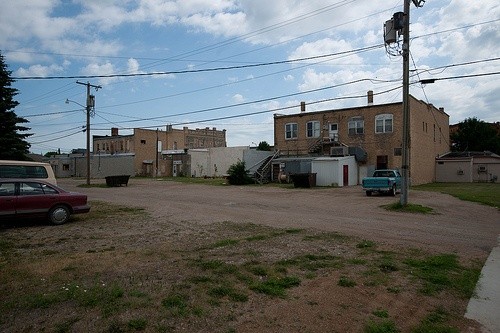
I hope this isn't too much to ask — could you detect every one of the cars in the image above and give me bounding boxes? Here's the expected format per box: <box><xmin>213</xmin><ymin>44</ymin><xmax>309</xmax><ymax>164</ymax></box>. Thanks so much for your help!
<box><xmin>0</xmin><ymin>180</ymin><xmax>92</xmax><ymax>225</ymax></box>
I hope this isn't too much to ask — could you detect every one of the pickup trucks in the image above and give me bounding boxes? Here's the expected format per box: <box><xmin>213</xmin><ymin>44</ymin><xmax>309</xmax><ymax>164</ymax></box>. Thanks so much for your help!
<box><xmin>361</xmin><ymin>170</ymin><xmax>403</xmax><ymax>196</ymax></box>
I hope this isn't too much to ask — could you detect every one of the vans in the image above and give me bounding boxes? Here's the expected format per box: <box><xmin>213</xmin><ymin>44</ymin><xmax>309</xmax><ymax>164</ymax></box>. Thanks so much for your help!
<box><xmin>0</xmin><ymin>161</ymin><xmax>58</xmax><ymax>187</ymax></box>
